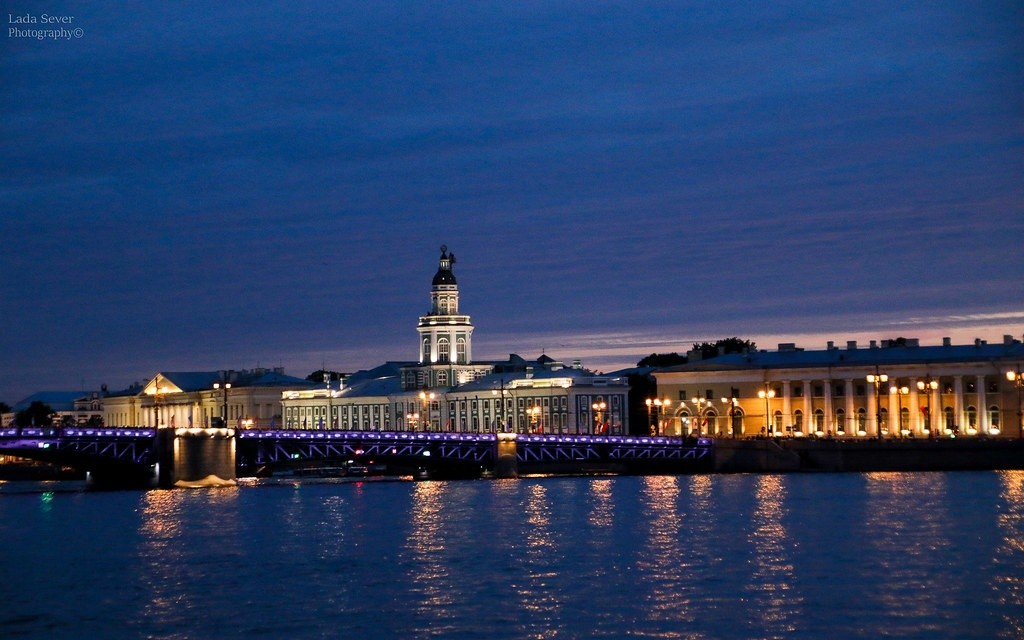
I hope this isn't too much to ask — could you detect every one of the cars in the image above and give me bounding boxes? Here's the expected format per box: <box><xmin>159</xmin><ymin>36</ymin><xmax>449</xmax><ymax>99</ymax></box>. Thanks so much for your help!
<box><xmin>338</xmin><ymin>454</ymin><xmax>376</xmax><ymax>478</ymax></box>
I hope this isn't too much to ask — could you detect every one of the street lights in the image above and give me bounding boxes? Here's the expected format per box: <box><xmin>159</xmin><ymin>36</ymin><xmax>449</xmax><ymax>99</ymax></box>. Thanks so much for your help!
<box><xmin>407</xmin><ymin>411</ymin><xmax>419</xmax><ymax>433</ymax></box>
<box><xmin>758</xmin><ymin>384</ymin><xmax>775</xmax><ymax>438</ymax></box>
<box><xmin>420</xmin><ymin>389</ymin><xmax>435</xmax><ymax>430</ymax></box>
<box><xmin>526</xmin><ymin>406</ymin><xmax>540</xmax><ymax>432</ymax></box>
<box><xmin>659</xmin><ymin>399</ymin><xmax>670</xmax><ymax>437</ymax></box>
<box><xmin>867</xmin><ymin>362</ymin><xmax>888</xmax><ymax>439</ymax></box>
<box><xmin>645</xmin><ymin>399</ymin><xmax>660</xmax><ymax>435</ymax></box>
<box><xmin>592</xmin><ymin>403</ymin><xmax>606</xmax><ymax>422</ymax></box>
<box><xmin>691</xmin><ymin>390</ymin><xmax>705</xmax><ymax>437</ymax></box>
<box><xmin>1006</xmin><ymin>362</ymin><xmax>1024</xmax><ymax>438</ymax></box>
<box><xmin>492</xmin><ymin>378</ymin><xmax>508</xmax><ymax>432</ymax></box>
<box><xmin>916</xmin><ymin>373</ymin><xmax>939</xmax><ymax>436</ymax></box>
<box><xmin>721</xmin><ymin>386</ymin><xmax>737</xmax><ymax>439</ymax></box>
<box><xmin>890</xmin><ymin>379</ymin><xmax>909</xmax><ymax>439</ymax></box>
<box><xmin>212</xmin><ymin>378</ymin><xmax>231</xmax><ymax>427</ymax></box>
